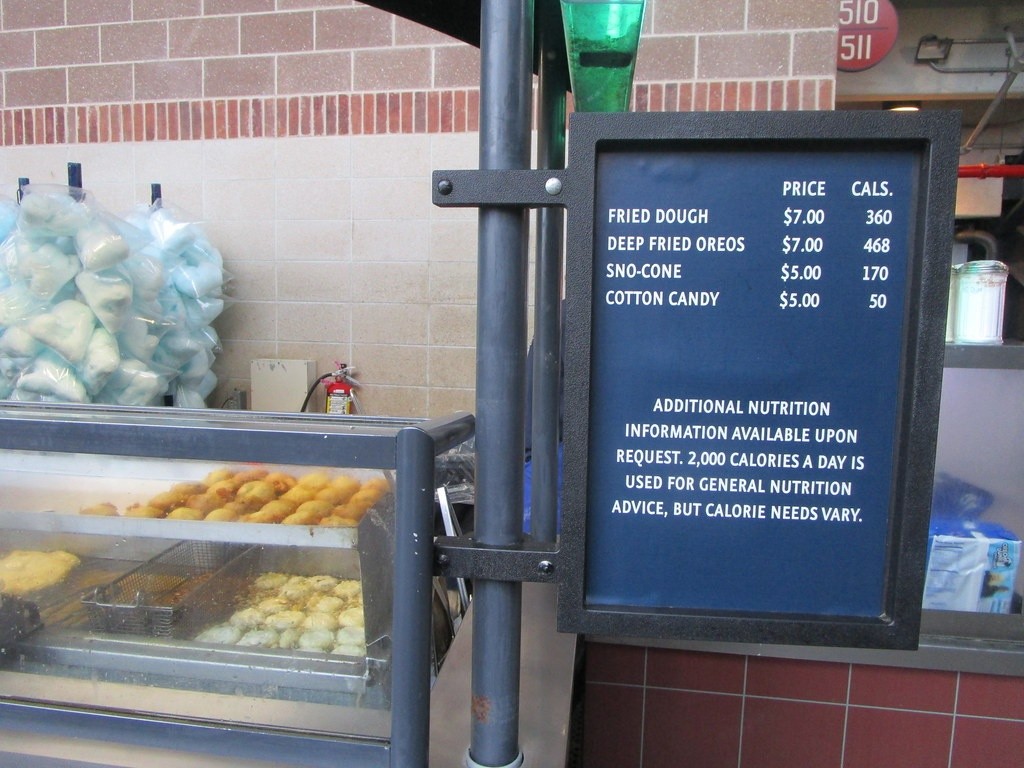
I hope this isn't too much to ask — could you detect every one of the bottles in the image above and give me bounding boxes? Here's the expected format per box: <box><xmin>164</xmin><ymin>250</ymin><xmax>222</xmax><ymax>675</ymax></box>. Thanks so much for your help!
<box><xmin>956</xmin><ymin>257</ymin><xmax>1009</xmax><ymax>344</ymax></box>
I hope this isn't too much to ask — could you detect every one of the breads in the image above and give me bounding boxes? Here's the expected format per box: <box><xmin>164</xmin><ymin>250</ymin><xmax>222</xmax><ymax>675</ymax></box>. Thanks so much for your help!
<box><xmin>0</xmin><ymin>196</ymin><xmax>225</xmax><ymax>403</ymax></box>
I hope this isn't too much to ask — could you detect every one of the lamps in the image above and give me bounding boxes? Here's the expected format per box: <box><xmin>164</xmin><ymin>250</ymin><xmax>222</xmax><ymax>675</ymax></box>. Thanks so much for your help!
<box><xmin>914</xmin><ymin>34</ymin><xmax>954</xmax><ymax>62</ymax></box>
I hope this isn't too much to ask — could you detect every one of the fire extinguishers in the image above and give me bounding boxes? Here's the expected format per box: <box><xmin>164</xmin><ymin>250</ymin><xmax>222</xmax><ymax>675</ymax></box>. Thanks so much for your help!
<box><xmin>301</xmin><ymin>360</ymin><xmax>359</xmax><ymax>414</ymax></box>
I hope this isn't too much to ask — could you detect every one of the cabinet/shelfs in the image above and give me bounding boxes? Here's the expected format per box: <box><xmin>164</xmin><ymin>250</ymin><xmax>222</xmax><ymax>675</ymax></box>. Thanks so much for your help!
<box><xmin>0</xmin><ymin>467</ymin><xmax>389</xmax><ymax>692</ymax></box>
